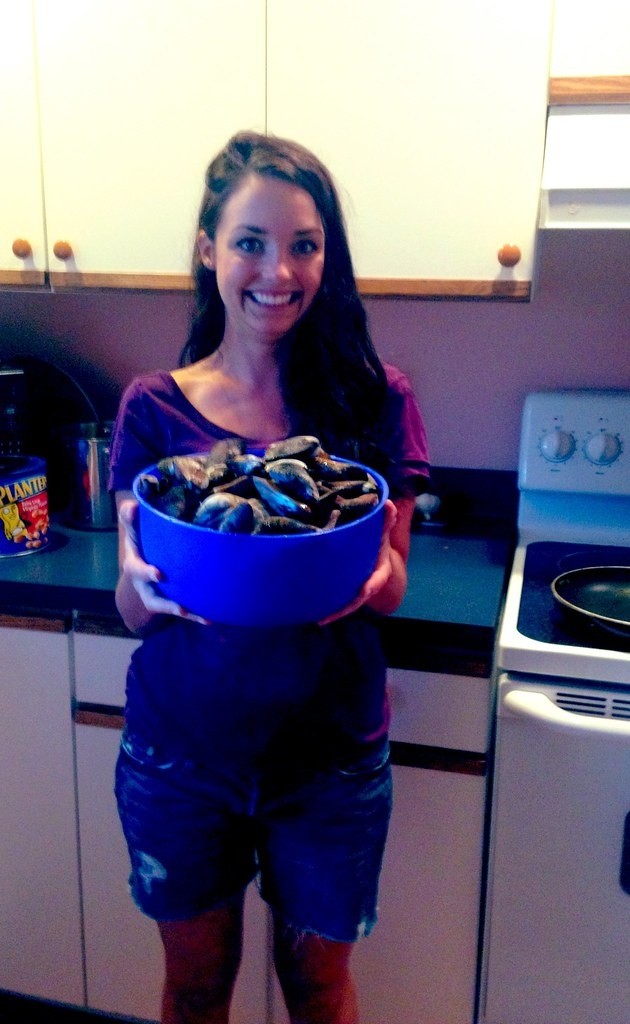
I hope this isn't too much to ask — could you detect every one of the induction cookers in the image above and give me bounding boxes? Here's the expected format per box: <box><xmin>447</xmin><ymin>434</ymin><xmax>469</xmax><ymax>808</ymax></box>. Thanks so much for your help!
<box><xmin>491</xmin><ymin>389</ymin><xmax>630</xmax><ymax>684</ymax></box>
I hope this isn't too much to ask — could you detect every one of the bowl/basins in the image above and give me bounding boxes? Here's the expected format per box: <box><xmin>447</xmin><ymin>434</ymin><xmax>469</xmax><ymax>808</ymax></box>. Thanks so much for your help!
<box><xmin>132</xmin><ymin>453</ymin><xmax>390</xmax><ymax>625</ymax></box>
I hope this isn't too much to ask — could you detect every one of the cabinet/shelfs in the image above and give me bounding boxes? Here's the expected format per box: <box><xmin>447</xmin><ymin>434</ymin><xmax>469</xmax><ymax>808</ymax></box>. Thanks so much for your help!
<box><xmin>0</xmin><ymin>0</ymin><xmax>556</xmax><ymax>298</ymax></box>
<box><xmin>0</xmin><ymin>608</ymin><xmax>492</xmax><ymax>1024</ymax></box>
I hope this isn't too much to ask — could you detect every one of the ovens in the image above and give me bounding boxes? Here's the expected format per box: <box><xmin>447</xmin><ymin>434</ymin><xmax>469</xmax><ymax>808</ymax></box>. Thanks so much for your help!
<box><xmin>478</xmin><ymin>672</ymin><xmax>630</xmax><ymax>1024</ymax></box>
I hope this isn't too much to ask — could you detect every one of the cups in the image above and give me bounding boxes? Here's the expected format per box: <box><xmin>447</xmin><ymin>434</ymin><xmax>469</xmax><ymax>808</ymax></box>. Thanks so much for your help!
<box><xmin>63</xmin><ymin>422</ymin><xmax>120</xmax><ymax>526</ymax></box>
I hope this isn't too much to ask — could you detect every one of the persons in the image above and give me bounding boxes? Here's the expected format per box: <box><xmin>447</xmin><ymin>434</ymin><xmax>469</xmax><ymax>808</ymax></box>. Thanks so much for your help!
<box><xmin>107</xmin><ymin>129</ymin><xmax>432</xmax><ymax>1024</ymax></box>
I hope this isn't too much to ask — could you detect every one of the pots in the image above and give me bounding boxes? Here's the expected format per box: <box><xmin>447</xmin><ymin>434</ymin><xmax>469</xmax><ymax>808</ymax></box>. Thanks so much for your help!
<box><xmin>549</xmin><ymin>552</ymin><xmax>630</xmax><ymax>634</ymax></box>
